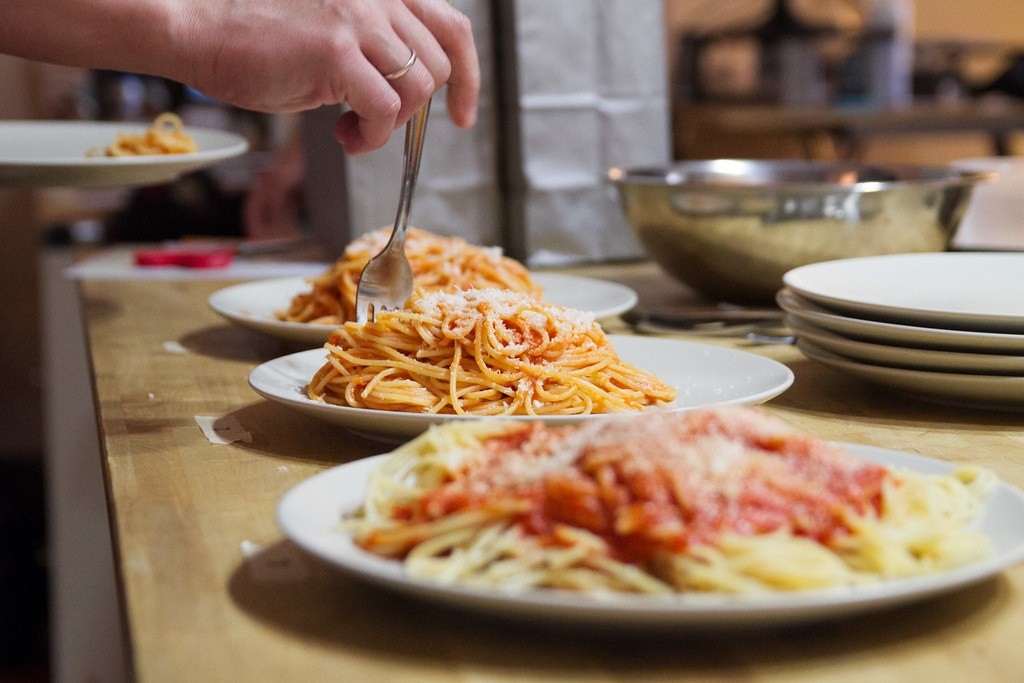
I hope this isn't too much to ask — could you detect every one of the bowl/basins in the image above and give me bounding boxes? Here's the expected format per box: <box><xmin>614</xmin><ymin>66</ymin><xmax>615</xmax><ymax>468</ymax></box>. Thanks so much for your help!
<box><xmin>598</xmin><ymin>162</ymin><xmax>998</xmax><ymax>298</ymax></box>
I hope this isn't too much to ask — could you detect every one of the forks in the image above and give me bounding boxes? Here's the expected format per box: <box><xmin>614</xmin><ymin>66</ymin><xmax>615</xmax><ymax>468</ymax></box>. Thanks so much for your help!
<box><xmin>357</xmin><ymin>97</ymin><xmax>429</xmax><ymax>322</ymax></box>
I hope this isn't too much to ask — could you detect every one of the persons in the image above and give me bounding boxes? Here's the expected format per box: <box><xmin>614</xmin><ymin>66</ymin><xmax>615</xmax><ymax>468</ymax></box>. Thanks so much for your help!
<box><xmin>2</xmin><ymin>0</ymin><xmax>480</xmax><ymax>158</ymax></box>
<box><xmin>134</xmin><ymin>75</ymin><xmax>247</xmax><ymax>240</ymax></box>
<box><xmin>247</xmin><ymin>1</ymin><xmax>674</xmax><ymax>265</ymax></box>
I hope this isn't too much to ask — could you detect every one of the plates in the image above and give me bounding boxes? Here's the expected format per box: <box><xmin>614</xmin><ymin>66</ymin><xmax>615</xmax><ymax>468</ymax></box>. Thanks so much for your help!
<box><xmin>275</xmin><ymin>439</ymin><xmax>1023</xmax><ymax>630</ymax></box>
<box><xmin>206</xmin><ymin>269</ymin><xmax>640</xmax><ymax>349</ymax></box>
<box><xmin>249</xmin><ymin>336</ymin><xmax>796</xmax><ymax>439</ymax></box>
<box><xmin>777</xmin><ymin>254</ymin><xmax>1023</xmax><ymax>401</ymax></box>
<box><xmin>1</xmin><ymin>120</ymin><xmax>248</xmax><ymax>188</ymax></box>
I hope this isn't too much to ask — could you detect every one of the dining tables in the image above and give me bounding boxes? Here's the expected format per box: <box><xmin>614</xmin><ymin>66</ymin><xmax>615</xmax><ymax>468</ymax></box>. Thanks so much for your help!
<box><xmin>36</xmin><ymin>236</ymin><xmax>1023</xmax><ymax>683</ymax></box>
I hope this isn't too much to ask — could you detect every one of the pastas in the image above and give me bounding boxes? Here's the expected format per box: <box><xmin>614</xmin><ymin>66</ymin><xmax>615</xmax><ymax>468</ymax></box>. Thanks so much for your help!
<box><xmin>307</xmin><ymin>282</ymin><xmax>673</xmax><ymax>419</ymax></box>
<box><xmin>102</xmin><ymin>112</ymin><xmax>197</xmax><ymax>159</ymax></box>
<box><xmin>277</xmin><ymin>222</ymin><xmax>539</xmax><ymax>325</ymax></box>
<box><xmin>344</xmin><ymin>413</ymin><xmax>991</xmax><ymax>600</ymax></box>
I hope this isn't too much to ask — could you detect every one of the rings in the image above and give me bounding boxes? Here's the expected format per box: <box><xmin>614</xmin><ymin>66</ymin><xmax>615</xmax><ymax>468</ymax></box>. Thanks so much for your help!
<box><xmin>382</xmin><ymin>46</ymin><xmax>417</xmax><ymax>80</ymax></box>
